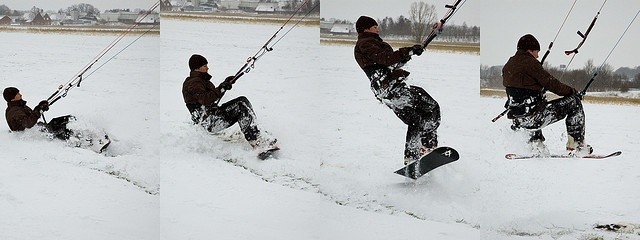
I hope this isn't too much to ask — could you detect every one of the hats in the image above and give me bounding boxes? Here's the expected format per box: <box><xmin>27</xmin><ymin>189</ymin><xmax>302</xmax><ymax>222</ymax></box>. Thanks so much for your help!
<box><xmin>3</xmin><ymin>87</ymin><xmax>19</xmax><ymax>102</ymax></box>
<box><xmin>356</xmin><ymin>16</ymin><xmax>378</xmax><ymax>34</ymax></box>
<box><xmin>189</xmin><ymin>55</ymin><xmax>208</xmax><ymax>72</ymax></box>
<box><xmin>517</xmin><ymin>34</ymin><xmax>540</xmax><ymax>50</ymax></box>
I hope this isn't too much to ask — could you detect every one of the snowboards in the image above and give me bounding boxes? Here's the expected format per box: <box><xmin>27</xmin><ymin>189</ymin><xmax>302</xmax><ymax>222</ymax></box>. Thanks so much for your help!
<box><xmin>86</xmin><ymin>134</ymin><xmax>112</xmax><ymax>152</ymax></box>
<box><xmin>258</xmin><ymin>139</ymin><xmax>280</xmax><ymax>160</ymax></box>
<box><xmin>394</xmin><ymin>146</ymin><xmax>460</xmax><ymax>179</ymax></box>
<box><xmin>505</xmin><ymin>151</ymin><xmax>621</xmax><ymax>159</ymax></box>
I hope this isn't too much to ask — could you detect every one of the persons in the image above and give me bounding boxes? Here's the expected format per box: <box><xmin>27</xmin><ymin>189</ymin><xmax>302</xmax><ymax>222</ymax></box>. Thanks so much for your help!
<box><xmin>354</xmin><ymin>16</ymin><xmax>441</xmax><ymax>165</ymax></box>
<box><xmin>3</xmin><ymin>87</ymin><xmax>92</xmax><ymax>148</ymax></box>
<box><xmin>182</xmin><ymin>54</ymin><xmax>280</xmax><ymax>160</ymax></box>
<box><xmin>502</xmin><ymin>34</ymin><xmax>593</xmax><ymax>157</ymax></box>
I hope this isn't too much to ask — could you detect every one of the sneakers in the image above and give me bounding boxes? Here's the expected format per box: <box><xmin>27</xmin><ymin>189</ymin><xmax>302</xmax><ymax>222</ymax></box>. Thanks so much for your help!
<box><xmin>568</xmin><ymin>142</ymin><xmax>593</xmax><ymax>155</ymax></box>
<box><xmin>532</xmin><ymin>140</ymin><xmax>550</xmax><ymax>155</ymax></box>
<box><xmin>404</xmin><ymin>158</ymin><xmax>414</xmax><ymax>165</ymax></box>
<box><xmin>419</xmin><ymin>146</ymin><xmax>433</xmax><ymax>156</ymax></box>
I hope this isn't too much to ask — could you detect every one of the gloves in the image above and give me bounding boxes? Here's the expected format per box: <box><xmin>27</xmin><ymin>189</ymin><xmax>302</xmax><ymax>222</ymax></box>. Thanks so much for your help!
<box><xmin>219</xmin><ymin>83</ymin><xmax>232</xmax><ymax>90</ymax></box>
<box><xmin>225</xmin><ymin>76</ymin><xmax>235</xmax><ymax>84</ymax></box>
<box><xmin>35</xmin><ymin>101</ymin><xmax>48</xmax><ymax>112</ymax></box>
<box><xmin>399</xmin><ymin>45</ymin><xmax>423</xmax><ymax>59</ymax></box>
